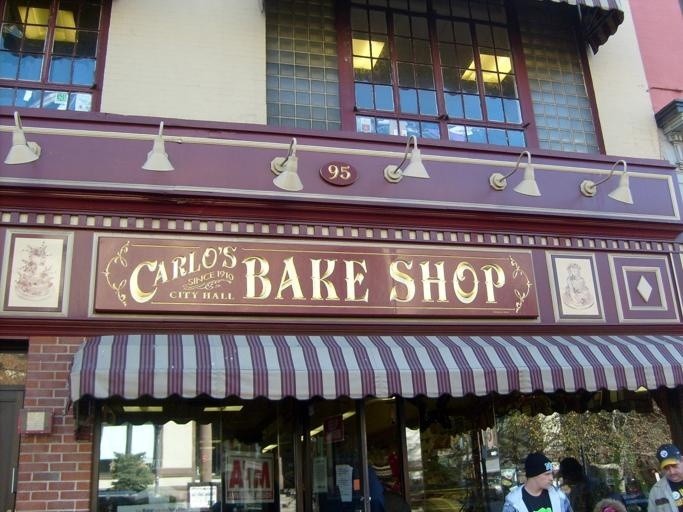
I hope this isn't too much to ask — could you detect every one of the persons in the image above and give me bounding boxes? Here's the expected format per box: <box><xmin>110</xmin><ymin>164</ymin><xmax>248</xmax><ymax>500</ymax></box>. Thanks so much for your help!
<box><xmin>557</xmin><ymin>456</ymin><xmax>608</xmax><ymax>511</ymax></box>
<box><xmin>502</xmin><ymin>449</ymin><xmax>573</xmax><ymax>511</ymax></box>
<box><xmin>335</xmin><ymin>434</ymin><xmax>383</xmax><ymax>512</ymax></box>
<box><xmin>199</xmin><ymin>470</ymin><xmax>243</xmax><ymax>511</ymax></box>
<box><xmin>648</xmin><ymin>443</ymin><xmax>683</xmax><ymax>510</ymax></box>
<box><xmin>129</xmin><ymin>468</ymin><xmax>186</xmax><ymax>511</ymax></box>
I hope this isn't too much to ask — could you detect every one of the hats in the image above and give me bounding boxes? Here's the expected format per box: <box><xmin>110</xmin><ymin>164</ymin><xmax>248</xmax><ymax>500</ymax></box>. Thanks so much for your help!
<box><xmin>525</xmin><ymin>453</ymin><xmax>553</xmax><ymax>478</ymax></box>
<box><xmin>657</xmin><ymin>444</ymin><xmax>681</xmax><ymax>469</ymax></box>
<box><xmin>555</xmin><ymin>457</ymin><xmax>582</xmax><ymax>478</ymax></box>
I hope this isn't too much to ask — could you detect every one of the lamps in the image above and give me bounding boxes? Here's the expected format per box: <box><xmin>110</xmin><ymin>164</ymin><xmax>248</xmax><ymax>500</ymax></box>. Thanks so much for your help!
<box><xmin>581</xmin><ymin>159</ymin><xmax>634</xmax><ymax>206</ymax></box>
<box><xmin>383</xmin><ymin>135</ymin><xmax>431</xmax><ymax>183</ymax></box>
<box><xmin>270</xmin><ymin>138</ymin><xmax>303</xmax><ymax>192</ymax></box>
<box><xmin>142</xmin><ymin>120</ymin><xmax>174</xmax><ymax>172</ymax></box>
<box><xmin>489</xmin><ymin>151</ymin><xmax>542</xmax><ymax>197</ymax></box>
<box><xmin>4</xmin><ymin>110</ymin><xmax>41</xmax><ymax>165</ymax></box>
